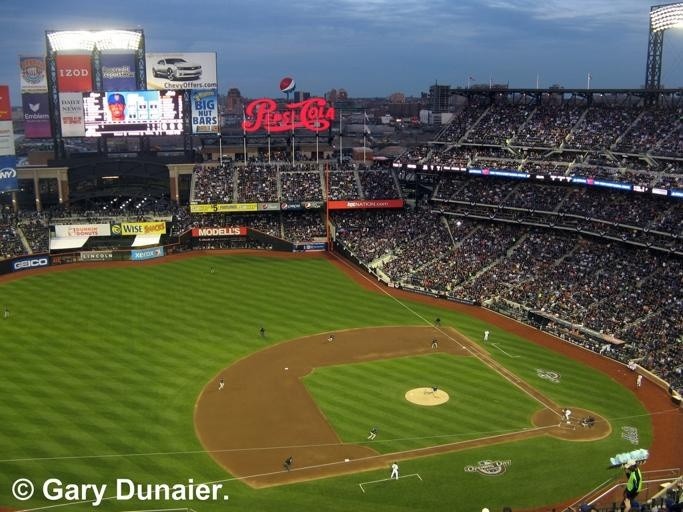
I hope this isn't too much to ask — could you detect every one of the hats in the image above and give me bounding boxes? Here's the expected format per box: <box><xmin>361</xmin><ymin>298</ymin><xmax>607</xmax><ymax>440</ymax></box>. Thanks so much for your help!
<box><xmin>581</xmin><ymin>503</ymin><xmax>591</xmax><ymax>512</ymax></box>
<box><xmin>625</xmin><ymin>460</ymin><xmax>635</xmax><ymax>468</ymax></box>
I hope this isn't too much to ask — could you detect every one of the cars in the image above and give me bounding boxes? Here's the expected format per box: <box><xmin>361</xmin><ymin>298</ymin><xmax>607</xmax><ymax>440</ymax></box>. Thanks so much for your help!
<box><xmin>152</xmin><ymin>55</ymin><xmax>203</xmax><ymax>82</ymax></box>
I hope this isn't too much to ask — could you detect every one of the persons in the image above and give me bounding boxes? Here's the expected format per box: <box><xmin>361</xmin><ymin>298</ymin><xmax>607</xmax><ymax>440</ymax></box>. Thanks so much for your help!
<box><xmin>107</xmin><ymin>94</ymin><xmax>125</xmax><ymax>120</ymax></box>
<box><xmin>284</xmin><ymin>455</ymin><xmax>295</xmax><ymax>471</ymax></box>
<box><xmin>558</xmin><ymin>408</ymin><xmax>576</xmax><ymax>431</ymax></box>
<box><xmin>587</xmin><ymin>508</ymin><xmax>599</xmax><ymax>512</ymax></box>
<box><xmin>368</xmin><ymin>428</ymin><xmax>377</xmax><ymax>440</ymax></box>
<box><xmin>665</xmin><ymin>479</ymin><xmax>683</xmax><ymax>512</ymax></box>
<box><xmin>503</xmin><ymin>506</ymin><xmax>512</xmax><ymax>512</ymax></box>
<box><xmin>621</xmin><ymin>459</ymin><xmax>643</xmax><ymax>505</ymax></box>
<box><xmin>390</xmin><ymin>461</ymin><xmax>400</xmax><ymax>480</ymax></box>
<box><xmin>623</xmin><ymin>498</ymin><xmax>632</xmax><ymax>512</ymax></box>
<box><xmin>481</xmin><ymin>507</ymin><xmax>490</xmax><ymax>512</ymax></box>
